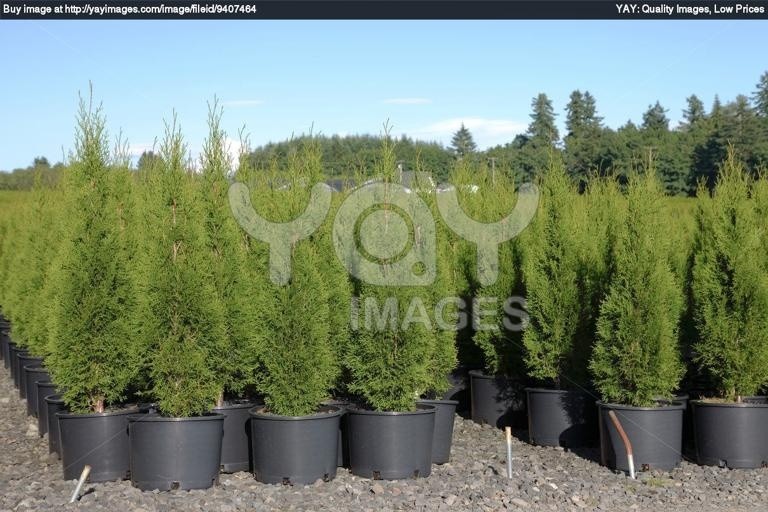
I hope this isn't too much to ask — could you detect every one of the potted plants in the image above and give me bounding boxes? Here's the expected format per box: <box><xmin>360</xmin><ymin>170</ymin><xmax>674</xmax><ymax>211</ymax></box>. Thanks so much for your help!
<box><xmin>0</xmin><ymin>76</ymin><xmax>768</xmax><ymax>494</ymax></box>
